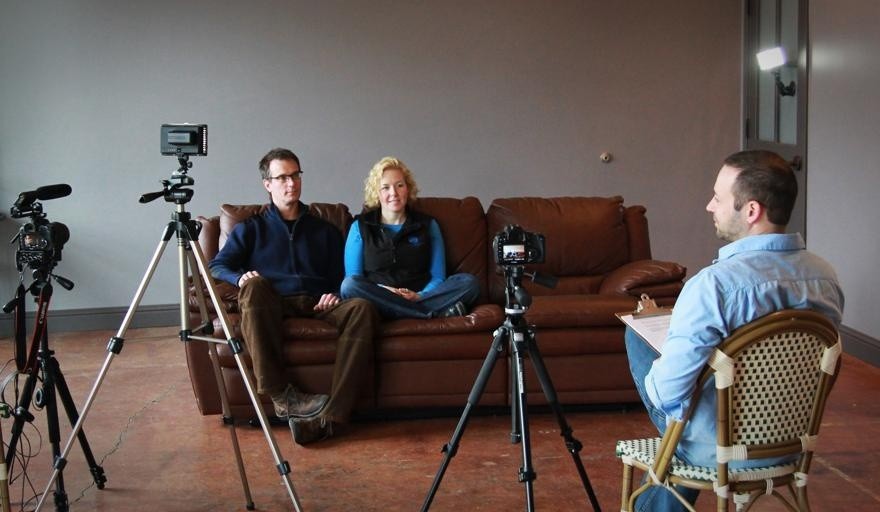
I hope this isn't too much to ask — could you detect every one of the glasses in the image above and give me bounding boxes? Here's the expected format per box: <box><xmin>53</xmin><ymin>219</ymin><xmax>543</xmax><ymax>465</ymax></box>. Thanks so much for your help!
<box><xmin>268</xmin><ymin>171</ymin><xmax>302</xmax><ymax>181</ymax></box>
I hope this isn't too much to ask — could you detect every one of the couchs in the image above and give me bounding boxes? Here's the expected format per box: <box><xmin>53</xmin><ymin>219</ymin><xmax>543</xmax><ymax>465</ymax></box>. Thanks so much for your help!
<box><xmin>174</xmin><ymin>190</ymin><xmax>689</xmax><ymax>432</ymax></box>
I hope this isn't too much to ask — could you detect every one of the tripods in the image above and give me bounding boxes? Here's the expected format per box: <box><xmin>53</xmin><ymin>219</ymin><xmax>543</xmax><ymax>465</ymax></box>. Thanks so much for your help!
<box><xmin>0</xmin><ymin>279</ymin><xmax>107</xmax><ymax>512</ymax></box>
<box><xmin>421</xmin><ymin>317</ymin><xmax>602</xmax><ymax>512</ymax></box>
<box><xmin>34</xmin><ymin>205</ymin><xmax>302</xmax><ymax>511</ymax></box>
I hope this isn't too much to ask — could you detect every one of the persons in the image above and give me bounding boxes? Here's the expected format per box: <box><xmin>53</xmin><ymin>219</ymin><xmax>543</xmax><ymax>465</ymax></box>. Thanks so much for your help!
<box><xmin>205</xmin><ymin>146</ymin><xmax>382</xmax><ymax>447</ymax></box>
<box><xmin>622</xmin><ymin>148</ymin><xmax>848</xmax><ymax>512</ymax></box>
<box><xmin>338</xmin><ymin>155</ymin><xmax>480</xmax><ymax>320</ymax></box>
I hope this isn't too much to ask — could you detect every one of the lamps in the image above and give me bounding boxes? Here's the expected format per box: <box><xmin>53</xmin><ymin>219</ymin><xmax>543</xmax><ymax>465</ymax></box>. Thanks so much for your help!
<box><xmin>754</xmin><ymin>41</ymin><xmax>798</xmax><ymax>102</ymax></box>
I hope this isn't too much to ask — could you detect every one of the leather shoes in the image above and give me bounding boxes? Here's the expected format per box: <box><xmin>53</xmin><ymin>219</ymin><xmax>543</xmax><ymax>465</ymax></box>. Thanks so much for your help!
<box><xmin>435</xmin><ymin>301</ymin><xmax>466</xmax><ymax>318</ymax></box>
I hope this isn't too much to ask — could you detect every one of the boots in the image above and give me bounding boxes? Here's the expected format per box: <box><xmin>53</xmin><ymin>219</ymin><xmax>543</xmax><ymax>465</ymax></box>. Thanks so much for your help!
<box><xmin>288</xmin><ymin>415</ymin><xmax>333</xmax><ymax>443</ymax></box>
<box><xmin>270</xmin><ymin>387</ymin><xmax>329</xmax><ymax>422</ymax></box>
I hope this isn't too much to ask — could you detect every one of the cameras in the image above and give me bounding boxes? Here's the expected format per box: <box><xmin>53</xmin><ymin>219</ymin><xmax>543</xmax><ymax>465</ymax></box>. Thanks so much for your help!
<box><xmin>492</xmin><ymin>223</ymin><xmax>546</xmax><ymax>267</ymax></box>
<box><xmin>16</xmin><ymin>223</ymin><xmax>70</xmax><ymax>268</ymax></box>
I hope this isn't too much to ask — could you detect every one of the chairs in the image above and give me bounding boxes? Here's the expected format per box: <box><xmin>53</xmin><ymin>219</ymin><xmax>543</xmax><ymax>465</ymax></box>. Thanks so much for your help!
<box><xmin>615</xmin><ymin>301</ymin><xmax>843</xmax><ymax>512</ymax></box>
